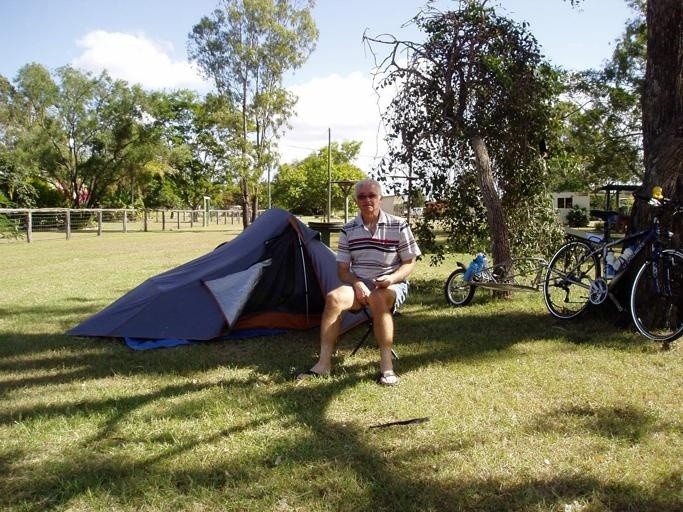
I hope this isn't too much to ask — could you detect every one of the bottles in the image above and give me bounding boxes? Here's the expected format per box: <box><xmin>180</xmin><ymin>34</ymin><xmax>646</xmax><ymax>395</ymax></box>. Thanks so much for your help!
<box><xmin>605</xmin><ymin>247</ymin><xmax>615</xmax><ymax>279</ymax></box>
<box><xmin>612</xmin><ymin>245</ymin><xmax>635</xmax><ymax>271</ymax></box>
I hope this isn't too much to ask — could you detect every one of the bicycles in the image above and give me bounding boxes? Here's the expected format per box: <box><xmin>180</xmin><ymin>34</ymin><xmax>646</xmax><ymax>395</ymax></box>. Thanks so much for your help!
<box><xmin>542</xmin><ymin>184</ymin><xmax>683</xmax><ymax>343</ymax></box>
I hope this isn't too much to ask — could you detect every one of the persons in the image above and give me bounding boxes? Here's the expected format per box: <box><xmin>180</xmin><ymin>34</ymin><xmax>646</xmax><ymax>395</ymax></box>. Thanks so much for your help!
<box><xmin>296</xmin><ymin>180</ymin><xmax>421</xmax><ymax>386</ymax></box>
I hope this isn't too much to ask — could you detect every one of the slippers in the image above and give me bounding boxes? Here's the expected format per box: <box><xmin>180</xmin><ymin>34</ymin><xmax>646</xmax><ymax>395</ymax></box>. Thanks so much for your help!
<box><xmin>295</xmin><ymin>370</ymin><xmax>322</xmax><ymax>381</ymax></box>
<box><xmin>380</xmin><ymin>372</ymin><xmax>399</xmax><ymax>387</ymax></box>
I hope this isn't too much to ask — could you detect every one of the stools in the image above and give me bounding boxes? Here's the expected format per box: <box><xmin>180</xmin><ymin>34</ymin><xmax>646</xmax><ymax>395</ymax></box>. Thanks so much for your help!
<box><xmin>348</xmin><ymin>303</ymin><xmax>399</xmax><ymax>362</ymax></box>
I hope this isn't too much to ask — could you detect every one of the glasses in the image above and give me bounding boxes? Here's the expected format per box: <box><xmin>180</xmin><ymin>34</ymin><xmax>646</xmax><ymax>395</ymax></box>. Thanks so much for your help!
<box><xmin>357</xmin><ymin>193</ymin><xmax>379</xmax><ymax>200</ymax></box>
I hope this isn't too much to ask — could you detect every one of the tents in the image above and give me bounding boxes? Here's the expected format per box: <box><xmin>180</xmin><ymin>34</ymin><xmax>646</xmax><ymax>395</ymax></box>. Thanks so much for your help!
<box><xmin>66</xmin><ymin>207</ymin><xmax>373</xmax><ymax>351</ymax></box>
